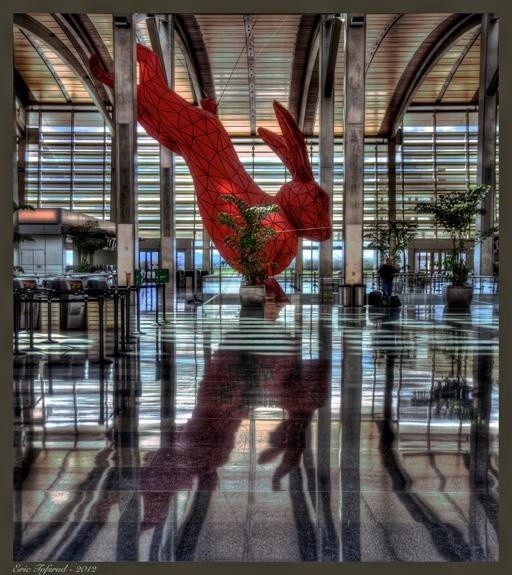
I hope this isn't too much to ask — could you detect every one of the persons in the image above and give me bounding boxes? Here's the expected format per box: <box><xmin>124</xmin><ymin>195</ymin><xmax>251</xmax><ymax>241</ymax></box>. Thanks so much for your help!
<box><xmin>376</xmin><ymin>258</ymin><xmax>398</xmax><ymax>308</ymax></box>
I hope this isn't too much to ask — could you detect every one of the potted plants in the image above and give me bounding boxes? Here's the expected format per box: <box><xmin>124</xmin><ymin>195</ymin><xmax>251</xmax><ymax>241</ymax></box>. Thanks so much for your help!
<box><xmin>212</xmin><ymin>193</ymin><xmax>281</xmax><ymax>308</ymax></box>
<box><xmin>412</xmin><ymin>183</ymin><xmax>499</xmax><ymax>312</ymax></box>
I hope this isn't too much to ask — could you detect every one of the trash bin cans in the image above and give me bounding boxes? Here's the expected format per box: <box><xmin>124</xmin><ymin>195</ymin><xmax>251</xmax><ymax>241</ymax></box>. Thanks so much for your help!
<box><xmin>338</xmin><ymin>284</ymin><xmax>366</xmax><ymax>307</ymax></box>
